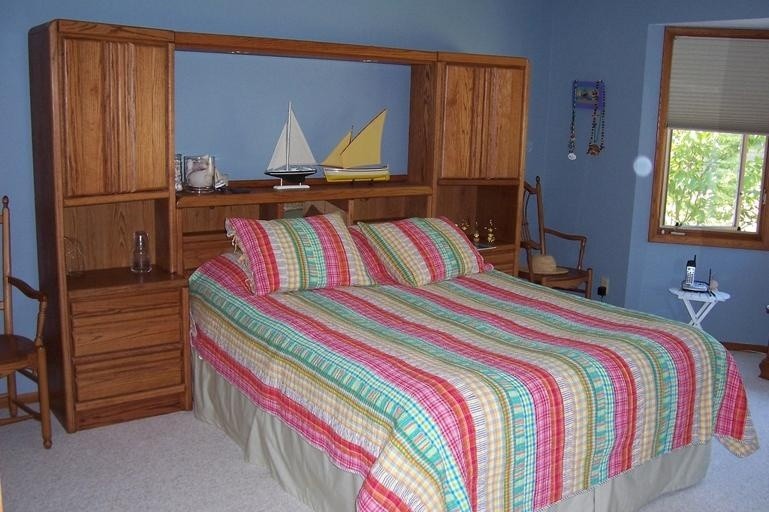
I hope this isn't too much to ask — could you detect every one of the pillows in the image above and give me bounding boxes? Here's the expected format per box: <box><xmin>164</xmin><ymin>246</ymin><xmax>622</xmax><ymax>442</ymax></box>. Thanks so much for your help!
<box><xmin>354</xmin><ymin>214</ymin><xmax>496</xmax><ymax>290</ymax></box>
<box><xmin>221</xmin><ymin>210</ymin><xmax>379</xmax><ymax>298</ymax></box>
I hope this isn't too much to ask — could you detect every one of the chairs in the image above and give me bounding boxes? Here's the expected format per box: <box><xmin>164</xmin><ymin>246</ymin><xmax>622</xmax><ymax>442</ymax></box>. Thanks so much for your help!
<box><xmin>518</xmin><ymin>175</ymin><xmax>593</xmax><ymax>300</ymax></box>
<box><xmin>0</xmin><ymin>193</ymin><xmax>53</xmax><ymax>449</ymax></box>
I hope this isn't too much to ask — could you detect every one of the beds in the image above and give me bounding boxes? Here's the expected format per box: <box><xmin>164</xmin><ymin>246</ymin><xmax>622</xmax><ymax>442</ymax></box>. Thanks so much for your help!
<box><xmin>188</xmin><ymin>248</ymin><xmax>722</xmax><ymax>510</ymax></box>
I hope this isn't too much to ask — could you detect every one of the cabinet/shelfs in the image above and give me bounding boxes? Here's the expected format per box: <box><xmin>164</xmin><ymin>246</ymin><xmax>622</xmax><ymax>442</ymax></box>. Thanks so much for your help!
<box><xmin>173</xmin><ymin>29</ymin><xmax>443</xmax><ymax>270</ymax></box>
<box><xmin>28</xmin><ymin>20</ymin><xmax>190</xmax><ymax>432</ymax></box>
<box><xmin>434</xmin><ymin>47</ymin><xmax>522</xmax><ymax>276</ymax></box>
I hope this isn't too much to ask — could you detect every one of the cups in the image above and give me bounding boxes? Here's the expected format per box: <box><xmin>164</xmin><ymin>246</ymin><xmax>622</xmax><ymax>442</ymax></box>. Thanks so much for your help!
<box><xmin>183</xmin><ymin>155</ymin><xmax>215</xmax><ymax>193</ymax></box>
<box><xmin>133</xmin><ymin>231</ymin><xmax>150</xmax><ymax>272</ymax></box>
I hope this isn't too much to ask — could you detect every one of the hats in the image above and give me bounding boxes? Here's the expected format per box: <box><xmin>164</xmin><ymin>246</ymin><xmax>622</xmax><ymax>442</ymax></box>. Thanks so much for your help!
<box><xmin>519</xmin><ymin>254</ymin><xmax>571</xmax><ymax>275</ymax></box>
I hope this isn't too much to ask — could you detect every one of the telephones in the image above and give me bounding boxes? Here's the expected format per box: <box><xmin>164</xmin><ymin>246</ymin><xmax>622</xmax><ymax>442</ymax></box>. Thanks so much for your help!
<box><xmin>682</xmin><ymin>255</ymin><xmax>709</xmax><ymax>293</ymax></box>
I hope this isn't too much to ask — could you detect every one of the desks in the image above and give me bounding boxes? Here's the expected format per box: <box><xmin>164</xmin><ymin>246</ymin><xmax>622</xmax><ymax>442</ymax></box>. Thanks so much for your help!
<box><xmin>669</xmin><ymin>284</ymin><xmax>730</xmax><ymax>333</ymax></box>
